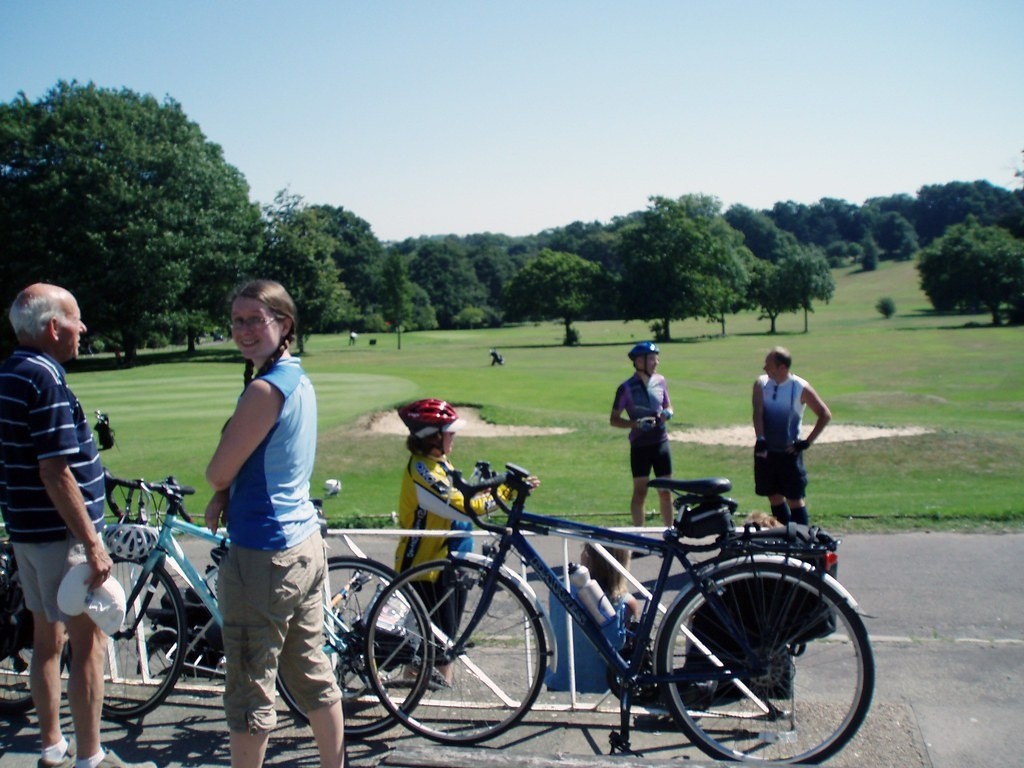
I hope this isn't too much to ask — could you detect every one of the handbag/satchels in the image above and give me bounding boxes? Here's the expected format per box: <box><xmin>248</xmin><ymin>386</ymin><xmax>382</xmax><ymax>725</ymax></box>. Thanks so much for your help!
<box><xmin>725</xmin><ymin>522</ymin><xmax>838</xmax><ymax>656</ymax></box>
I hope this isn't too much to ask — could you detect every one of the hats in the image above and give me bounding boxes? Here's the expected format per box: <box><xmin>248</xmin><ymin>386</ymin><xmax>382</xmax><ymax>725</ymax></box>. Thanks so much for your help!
<box><xmin>57</xmin><ymin>561</ymin><xmax>126</xmax><ymax>636</ymax></box>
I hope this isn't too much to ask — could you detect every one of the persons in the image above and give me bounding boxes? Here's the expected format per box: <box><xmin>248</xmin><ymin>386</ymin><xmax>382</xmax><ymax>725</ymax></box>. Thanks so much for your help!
<box><xmin>350</xmin><ymin>330</ymin><xmax>357</xmax><ymax>345</ymax></box>
<box><xmin>490</xmin><ymin>351</ymin><xmax>503</xmax><ymax>365</ymax></box>
<box><xmin>394</xmin><ymin>399</ymin><xmax>541</xmax><ymax>687</ymax></box>
<box><xmin>609</xmin><ymin>342</ymin><xmax>675</xmax><ymax>527</ymax></box>
<box><xmin>0</xmin><ymin>282</ymin><xmax>156</xmax><ymax>768</ymax></box>
<box><xmin>204</xmin><ymin>280</ymin><xmax>346</xmax><ymax>768</ymax></box>
<box><xmin>579</xmin><ymin>544</ymin><xmax>640</xmax><ymax>622</ymax></box>
<box><xmin>741</xmin><ymin>510</ymin><xmax>784</xmax><ymax>528</ymax></box>
<box><xmin>753</xmin><ymin>347</ymin><xmax>832</xmax><ymax>526</ymax></box>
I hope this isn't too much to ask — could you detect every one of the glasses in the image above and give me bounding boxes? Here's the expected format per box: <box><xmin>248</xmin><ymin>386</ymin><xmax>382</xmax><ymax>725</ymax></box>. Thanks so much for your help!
<box><xmin>232</xmin><ymin>316</ymin><xmax>277</xmax><ymax>328</ymax></box>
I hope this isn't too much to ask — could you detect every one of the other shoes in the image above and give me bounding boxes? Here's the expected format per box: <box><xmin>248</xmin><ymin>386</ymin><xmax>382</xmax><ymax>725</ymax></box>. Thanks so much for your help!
<box><xmin>403</xmin><ymin>666</ymin><xmax>418</xmax><ymax>679</ymax></box>
<box><xmin>72</xmin><ymin>749</ymin><xmax>157</xmax><ymax>768</ymax></box>
<box><xmin>37</xmin><ymin>735</ymin><xmax>76</xmax><ymax>768</ymax></box>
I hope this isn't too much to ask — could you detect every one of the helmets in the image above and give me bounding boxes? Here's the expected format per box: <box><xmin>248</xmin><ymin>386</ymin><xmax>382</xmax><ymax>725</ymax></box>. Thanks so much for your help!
<box><xmin>102</xmin><ymin>523</ymin><xmax>160</xmax><ymax>560</ymax></box>
<box><xmin>628</xmin><ymin>340</ymin><xmax>660</xmax><ymax>360</ymax></box>
<box><xmin>397</xmin><ymin>398</ymin><xmax>459</xmax><ymax>428</ymax></box>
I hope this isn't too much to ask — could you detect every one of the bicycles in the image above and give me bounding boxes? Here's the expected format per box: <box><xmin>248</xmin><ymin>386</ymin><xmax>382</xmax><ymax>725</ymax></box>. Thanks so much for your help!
<box><xmin>362</xmin><ymin>459</ymin><xmax>874</xmax><ymax>765</ymax></box>
<box><xmin>68</xmin><ymin>466</ymin><xmax>434</xmax><ymax>737</ymax></box>
<box><xmin>0</xmin><ymin>542</ymin><xmax>64</xmax><ymax>714</ymax></box>
